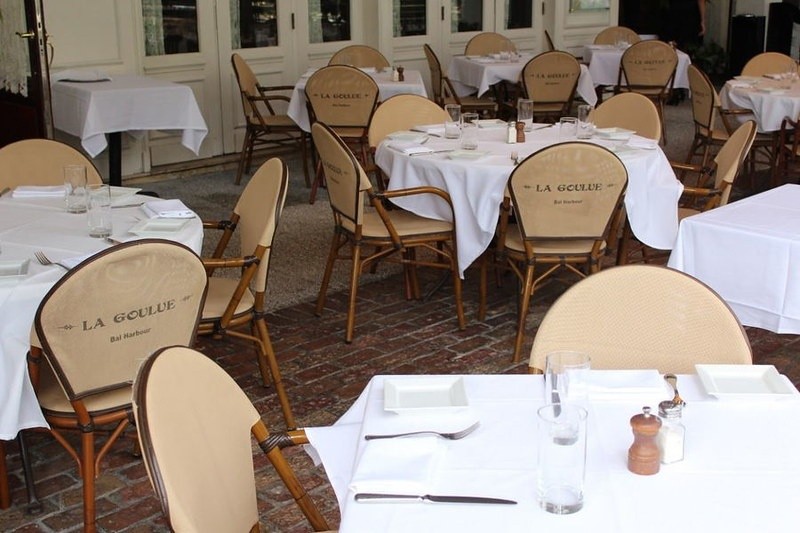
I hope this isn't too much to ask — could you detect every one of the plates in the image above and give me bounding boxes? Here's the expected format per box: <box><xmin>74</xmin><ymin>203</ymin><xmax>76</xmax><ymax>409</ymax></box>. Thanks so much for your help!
<box><xmin>92</xmin><ymin>184</ymin><xmax>191</xmax><ymax>235</ymax></box>
<box><xmin>695</xmin><ymin>364</ymin><xmax>794</xmax><ymax>402</ymax></box>
<box><xmin>470</xmin><ymin>118</ymin><xmax>508</xmax><ymax>129</ymax></box>
<box><xmin>447</xmin><ymin>148</ymin><xmax>490</xmax><ymax>161</ymax></box>
<box><xmin>382</xmin><ymin>377</ymin><xmax>469</xmax><ymax>416</ymax></box>
<box><xmin>386</xmin><ymin>130</ymin><xmax>427</xmax><ymax>140</ymax></box>
<box><xmin>595</xmin><ymin>126</ymin><xmax>636</xmax><ymax>138</ymax></box>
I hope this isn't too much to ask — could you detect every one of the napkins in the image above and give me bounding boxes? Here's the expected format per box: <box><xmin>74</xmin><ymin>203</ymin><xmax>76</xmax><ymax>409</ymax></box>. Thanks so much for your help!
<box><xmin>626</xmin><ymin>137</ymin><xmax>659</xmax><ymax>150</ymax></box>
<box><xmin>565</xmin><ymin>369</ymin><xmax>668</xmax><ymax>399</ymax></box>
<box><xmin>388</xmin><ymin>143</ymin><xmax>432</xmax><ymax>154</ymax></box>
<box><xmin>12</xmin><ymin>186</ymin><xmax>66</xmax><ymax>198</ymax></box>
<box><xmin>759</xmin><ymin>87</ymin><xmax>786</xmax><ymax>96</ymax></box>
<box><xmin>727</xmin><ymin>81</ymin><xmax>750</xmax><ymax>88</ymax></box>
<box><xmin>348</xmin><ymin>437</ymin><xmax>438</xmax><ymax>494</ymax></box>
<box><xmin>140</xmin><ymin>199</ymin><xmax>196</xmax><ymax>219</ymax></box>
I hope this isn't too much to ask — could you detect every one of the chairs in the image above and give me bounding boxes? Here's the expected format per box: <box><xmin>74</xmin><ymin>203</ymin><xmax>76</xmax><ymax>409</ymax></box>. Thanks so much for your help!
<box><xmin>0</xmin><ymin>26</ymin><xmax>800</xmax><ymax>533</ymax></box>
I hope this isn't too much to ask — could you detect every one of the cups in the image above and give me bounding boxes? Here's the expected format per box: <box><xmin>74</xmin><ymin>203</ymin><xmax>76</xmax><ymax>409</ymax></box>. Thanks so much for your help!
<box><xmin>536</xmin><ymin>404</ymin><xmax>589</xmax><ymax>514</ymax></box>
<box><xmin>460</xmin><ymin>113</ymin><xmax>479</xmax><ymax>149</ymax></box>
<box><xmin>445</xmin><ymin>103</ymin><xmax>460</xmax><ymax>139</ymax></box>
<box><xmin>559</xmin><ymin>117</ymin><xmax>578</xmax><ymax>142</ymax></box>
<box><xmin>578</xmin><ymin>104</ymin><xmax>593</xmax><ymax>139</ymax></box>
<box><xmin>544</xmin><ymin>350</ymin><xmax>592</xmax><ymax>418</ymax></box>
<box><xmin>62</xmin><ymin>164</ymin><xmax>113</xmax><ymax>239</ymax></box>
<box><xmin>517</xmin><ymin>99</ymin><xmax>534</xmax><ymax>131</ymax></box>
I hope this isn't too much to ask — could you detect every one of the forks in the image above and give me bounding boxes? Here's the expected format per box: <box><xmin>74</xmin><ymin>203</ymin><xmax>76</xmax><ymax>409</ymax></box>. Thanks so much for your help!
<box><xmin>364</xmin><ymin>421</ymin><xmax>479</xmax><ymax>439</ymax></box>
<box><xmin>664</xmin><ymin>373</ymin><xmax>686</xmax><ymax>406</ymax></box>
<box><xmin>34</xmin><ymin>251</ymin><xmax>70</xmax><ymax>271</ymax></box>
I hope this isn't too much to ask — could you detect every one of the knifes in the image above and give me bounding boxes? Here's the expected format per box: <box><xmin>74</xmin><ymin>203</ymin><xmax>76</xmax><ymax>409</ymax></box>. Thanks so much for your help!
<box><xmin>103</xmin><ymin>235</ymin><xmax>121</xmax><ymax>245</ymax></box>
<box><xmin>355</xmin><ymin>493</ymin><xmax>518</xmax><ymax>506</ymax></box>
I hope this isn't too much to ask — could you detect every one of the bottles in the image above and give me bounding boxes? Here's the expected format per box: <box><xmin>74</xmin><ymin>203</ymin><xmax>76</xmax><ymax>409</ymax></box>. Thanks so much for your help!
<box><xmin>657</xmin><ymin>401</ymin><xmax>686</xmax><ymax>464</ymax></box>
<box><xmin>390</xmin><ymin>64</ymin><xmax>404</xmax><ymax>81</ymax></box>
<box><xmin>507</xmin><ymin>121</ymin><xmax>516</xmax><ymax>142</ymax></box>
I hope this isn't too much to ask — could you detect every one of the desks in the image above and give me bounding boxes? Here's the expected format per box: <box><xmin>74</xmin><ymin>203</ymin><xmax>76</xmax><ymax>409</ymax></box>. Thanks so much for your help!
<box><xmin>583</xmin><ymin>43</ymin><xmax>693</xmax><ymax>108</ymax></box>
<box><xmin>0</xmin><ymin>186</ymin><xmax>204</xmax><ymax>510</ymax></box>
<box><xmin>717</xmin><ymin>80</ymin><xmax>800</xmax><ymax>183</ymax></box>
<box><xmin>298</xmin><ymin>374</ymin><xmax>800</xmax><ymax>533</ymax></box>
<box><xmin>50</xmin><ymin>73</ymin><xmax>209</xmax><ymax>199</ymax></box>
<box><xmin>287</xmin><ymin>67</ymin><xmax>428</xmax><ymax>188</ymax></box>
<box><xmin>444</xmin><ymin>53</ymin><xmax>598</xmax><ymax>122</ymax></box>
<box><xmin>374</xmin><ymin>119</ymin><xmax>684</xmax><ymax>321</ymax></box>
<box><xmin>668</xmin><ymin>183</ymin><xmax>800</xmax><ymax>335</ymax></box>
